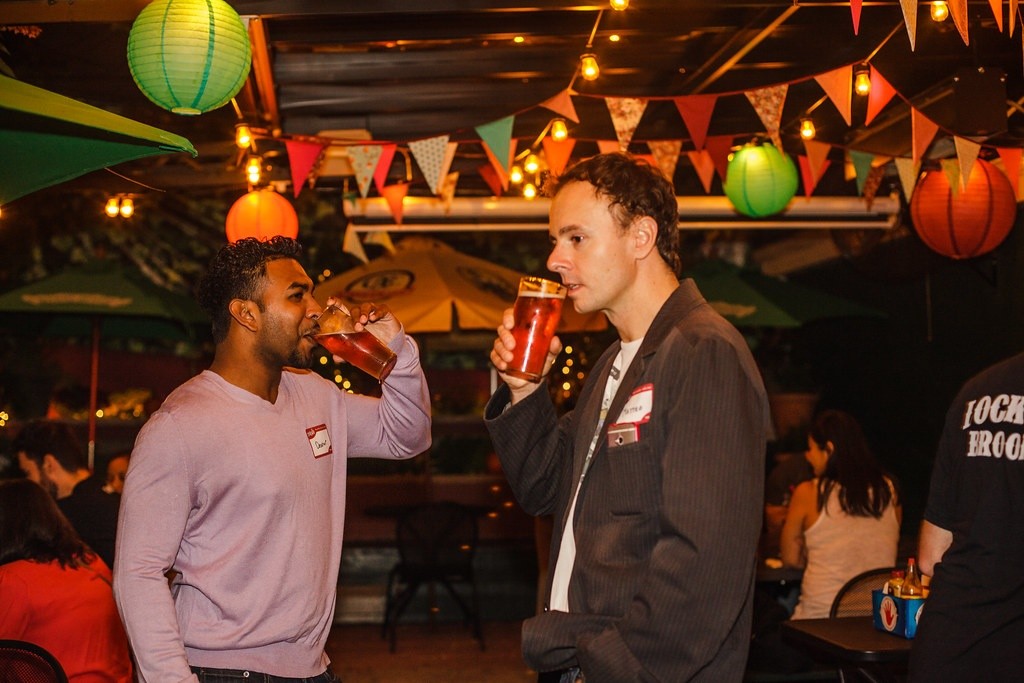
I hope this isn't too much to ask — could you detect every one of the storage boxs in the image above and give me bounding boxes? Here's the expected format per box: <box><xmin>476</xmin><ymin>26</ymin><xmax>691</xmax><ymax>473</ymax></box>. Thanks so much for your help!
<box><xmin>872</xmin><ymin>588</ymin><xmax>926</xmax><ymax>638</ymax></box>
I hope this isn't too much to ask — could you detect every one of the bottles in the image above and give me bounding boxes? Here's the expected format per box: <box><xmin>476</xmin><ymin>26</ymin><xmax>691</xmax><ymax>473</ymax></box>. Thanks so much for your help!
<box><xmin>888</xmin><ymin>569</ymin><xmax>905</xmax><ymax>598</ymax></box>
<box><xmin>901</xmin><ymin>557</ymin><xmax>922</xmax><ymax>599</ymax></box>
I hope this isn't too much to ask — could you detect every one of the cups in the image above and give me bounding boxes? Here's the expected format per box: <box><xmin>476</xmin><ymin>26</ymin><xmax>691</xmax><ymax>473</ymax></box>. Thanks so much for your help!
<box><xmin>309</xmin><ymin>306</ymin><xmax>397</xmax><ymax>382</ymax></box>
<box><xmin>498</xmin><ymin>275</ymin><xmax>567</xmax><ymax>383</ymax></box>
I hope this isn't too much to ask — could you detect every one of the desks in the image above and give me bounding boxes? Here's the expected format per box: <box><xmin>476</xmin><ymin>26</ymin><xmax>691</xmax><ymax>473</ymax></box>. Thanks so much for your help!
<box><xmin>20</xmin><ymin>417</ymin><xmax>148</xmax><ymax>462</ymax></box>
<box><xmin>754</xmin><ymin>534</ymin><xmax>918</xmax><ymax>615</ymax></box>
<box><xmin>779</xmin><ymin>615</ymin><xmax>913</xmax><ymax>683</ymax></box>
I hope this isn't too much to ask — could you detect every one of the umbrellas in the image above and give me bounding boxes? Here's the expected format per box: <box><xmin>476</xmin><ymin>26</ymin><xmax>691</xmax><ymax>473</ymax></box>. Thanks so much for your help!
<box><xmin>310</xmin><ymin>236</ymin><xmax>605</xmax><ymax>349</ymax></box>
<box><xmin>0</xmin><ymin>245</ymin><xmax>217</xmax><ymax>471</ymax></box>
<box><xmin>1</xmin><ymin>61</ymin><xmax>196</xmax><ymax>211</ymax></box>
<box><xmin>687</xmin><ymin>257</ymin><xmax>890</xmax><ymax>327</ymax></box>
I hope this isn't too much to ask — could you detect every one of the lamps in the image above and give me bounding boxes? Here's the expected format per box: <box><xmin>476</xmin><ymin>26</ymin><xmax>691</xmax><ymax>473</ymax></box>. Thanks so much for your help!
<box><xmin>335</xmin><ymin>176</ymin><xmax>900</xmax><ymax>235</ymax></box>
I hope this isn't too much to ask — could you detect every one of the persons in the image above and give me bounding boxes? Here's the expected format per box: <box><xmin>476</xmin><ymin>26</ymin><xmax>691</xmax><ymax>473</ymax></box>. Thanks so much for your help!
<box><xmin>113</xmin><ymin>236</ymin><xmax>432</xmax><ymax>683</ymax></box>
<box><xmin>483</xmin><ymin>151</ymin><xmax>768</xmax><ymax>683</ymax></box>
<box><xmin>751</xmin><ymin>410</ymin><xmax>901</xmax><ymax>673</ymax></box>
<box><xmin>906</xmin><ymin>351</ymin><xmax>1024</xmax><ymax>683</ymax></box>
<box><xmin>0</xmin><ymin>421</ymin><xmax>139</xmax><ymax>683</ymax></box>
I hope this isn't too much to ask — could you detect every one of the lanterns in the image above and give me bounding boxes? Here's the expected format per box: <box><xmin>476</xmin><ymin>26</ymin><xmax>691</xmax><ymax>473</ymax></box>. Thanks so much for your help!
<box><xmin>126</xmin><ymin>0</ymin><xmax>252</xmax><ymax>115</ymax></box>
<box><xmin>911</xmin><ymin>158</ymin><xmax>1016</xmax><ymax>259</ymax></box>
<box><xmin>227</xmin><ymin>188</ymin><xmax>299</xmax><ymax>244</ymax></box>
<box><xmin>721</xmin><ymin>142</ymin><xmax>798</xmax><ymax>216</ymax></box>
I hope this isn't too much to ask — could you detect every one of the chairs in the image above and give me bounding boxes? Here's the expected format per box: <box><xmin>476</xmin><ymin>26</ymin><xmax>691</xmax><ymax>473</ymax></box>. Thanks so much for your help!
<box><xmin>380</xmin><ymin>502</ymin><xmax>485</xmax><ymax>653</ymax></box>
<box><xmin>829</xmin><ymin>566</ymin><xmax>923</xmax><ymax>683</ymax></box>
